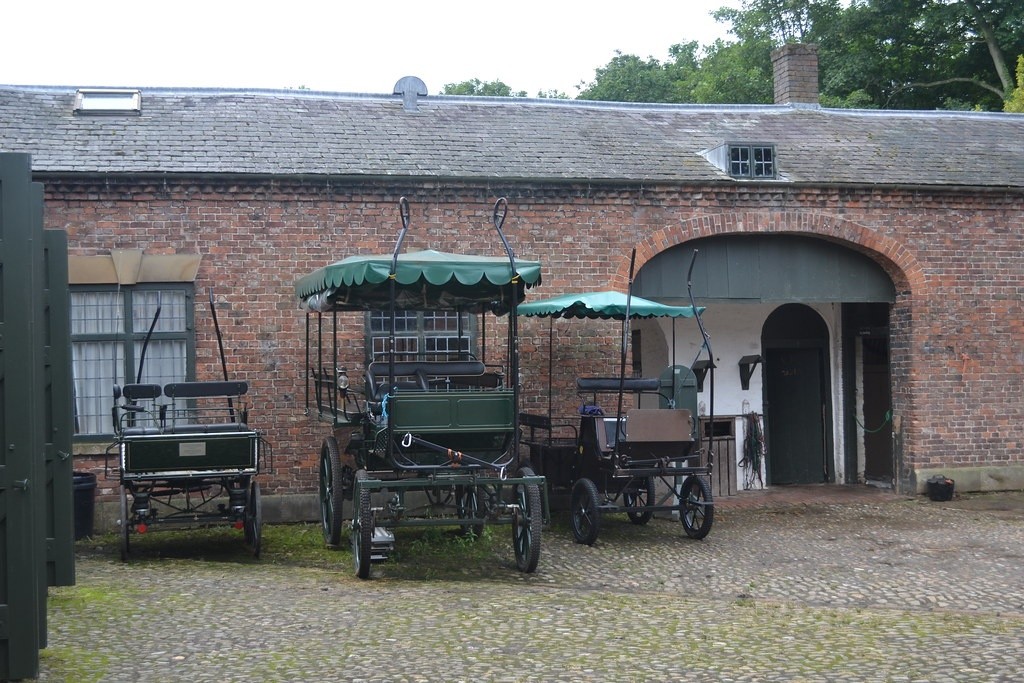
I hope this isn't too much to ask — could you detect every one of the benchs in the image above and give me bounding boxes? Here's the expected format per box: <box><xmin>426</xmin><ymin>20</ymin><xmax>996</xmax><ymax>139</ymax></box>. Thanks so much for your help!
<box><xmin>112</xmin><ymin>380</ymin><xmax>250</xmax><ymax>436</ymax></box>
<box><xmin>574</xmin><ymin>376</ymin><xmax>678</xmax><ymax>419</ymax></box>
<box><xmin>364</xmin><ymin>359</ymin><xmax>505</xmax><ymax>416</ymax></box>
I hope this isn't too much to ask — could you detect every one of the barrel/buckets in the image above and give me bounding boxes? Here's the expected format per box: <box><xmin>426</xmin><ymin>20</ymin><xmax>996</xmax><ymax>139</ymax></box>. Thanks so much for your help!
<box><xmin>928</xmin><ymin>473</ymin><xmax>954</xmax><ymax>502</ymax></box>
<box><xmin>72</xmin><ymin>470</ymin><xmax>98</xmax><ymax>540</ymax></box>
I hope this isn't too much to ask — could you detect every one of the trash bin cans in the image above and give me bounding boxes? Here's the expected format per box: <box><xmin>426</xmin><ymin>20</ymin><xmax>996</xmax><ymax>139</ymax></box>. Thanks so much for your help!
<box><xmin>687</xmin><ymin>415</ymin><xmax>739</xmax><ymax>498</ymax></box>
<box><xmin>72</xmin><ymin>471</ymin><xmax>97</xmax><ymax>542</ymax></box>
<box><xmin>927</xmin><ymin>473</ymin><xmax>955</xmax><ymax>502</ymax></box>
